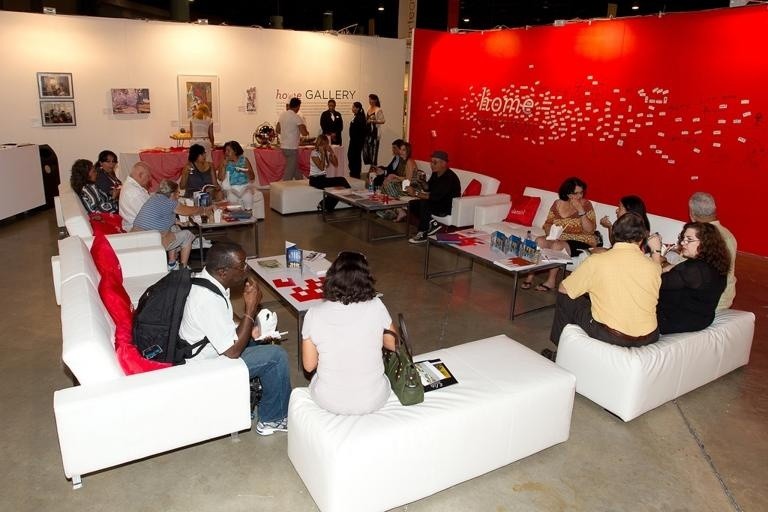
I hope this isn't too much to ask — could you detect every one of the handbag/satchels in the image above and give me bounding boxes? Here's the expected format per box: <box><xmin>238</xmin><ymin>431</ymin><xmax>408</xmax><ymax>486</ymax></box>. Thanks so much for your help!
<box><xmin>383</xmin><ymin>313</ymin><xmax>424</xmax><ymax>407</ymax></box>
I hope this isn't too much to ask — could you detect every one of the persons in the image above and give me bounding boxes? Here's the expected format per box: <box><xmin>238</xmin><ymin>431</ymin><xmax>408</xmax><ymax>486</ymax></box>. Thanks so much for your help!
<box><xmin>590</xmin><ymin>191</ymin><xmax>651</xmax><ymax>258</ymax></box>
<box><xmin>520</xmin><ymin>175</ymin><xmax>603</xmax><ymax>291</ymax></box>
<box><xmin>176</xmin><ymin>236</ymin><xmax>294</xmax><ymax>435</ymax></box>
<box><xmin>655</xmin><ymin>222</ymin><xmax>731</xmax><ymax>334</ymax></box>
<box><xmin>116</xmin><ymin>161</ymin><xmax>155</xmax><ymax>230</ymax></box>
<box><xmin>92</xmin><ymin>151</ymin><xmax>125</xmax><ymax>199</ymax></box>
<box><xmin>297</xmin><ymin>249</ymin><xmax>395</xmax><ymax>417</ymax></box>
<box><xmin>68</xmin><ymin>159</ymin><xmax>123</xmax><ymax>216</ymax></box>
<box><xmin>123</xmin><ymin>178</ymin><xmax>217</xmax><ymax>272</ymax></box>
<box><xmin>678</xmin><ymin>186</ymin><xmax>738</xmax><ymax>317</ymax></box>
<box><xmin>540</xmin><ymin>213</ymin><xmax>662</xmax><ymax>361</ymax></box>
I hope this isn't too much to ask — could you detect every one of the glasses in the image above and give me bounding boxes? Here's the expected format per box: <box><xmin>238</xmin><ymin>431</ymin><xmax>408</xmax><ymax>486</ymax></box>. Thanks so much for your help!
<box><xmin>231</xmin><ymin>263</ymin><xmax>248</xmax><ymax>273</ymax></box>
<box><xmin>678</xmin><ymin>235</ymin><xmax>698</xmax><ymax>242</ymax></box>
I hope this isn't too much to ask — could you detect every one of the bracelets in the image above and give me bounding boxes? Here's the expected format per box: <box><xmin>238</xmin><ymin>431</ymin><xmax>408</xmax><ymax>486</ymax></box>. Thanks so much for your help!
<box><xmin>242</xmin><ymin>311</ymin><xmax>255</xmax><ymax>326</ymax></box>
<box><xmin>578</xmin><ymin>212</ymin><xmax>588</xmax><ymax>217</ymax></box>
<box><xmin>202</xmin><ymin>205</ymin><xmax>206</xmax><ymax>216</ymax></box>
<box><xmin>649</xmin><ymin>249</ymin><xmax>664</xmax><ymax>256</ymax></box>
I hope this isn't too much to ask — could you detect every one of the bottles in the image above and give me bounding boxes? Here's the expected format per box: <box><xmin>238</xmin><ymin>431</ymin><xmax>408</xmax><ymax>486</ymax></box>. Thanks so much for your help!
<box><xmin>192</xmin><ymin>190</ymin><xmax>223</xmax><ymax>223</ymax></box>
<box><xmin>366</xmin><ymin>183</ymin><xmax>392</xmax><ymax>203</ymax></box>
<box><xmin>413</xmin><ymin>168</ymin><xmax>426</xmax><ymax>184</ymax></box>
<box><xmin>401</xmin><ymin>177</ymin><xmax>411</xmax><ymax>191</ymax></box>
<box><xmin>527</xmin><ymin>230</ymin><xmax>534</xmax><ymax>240</ymax></box>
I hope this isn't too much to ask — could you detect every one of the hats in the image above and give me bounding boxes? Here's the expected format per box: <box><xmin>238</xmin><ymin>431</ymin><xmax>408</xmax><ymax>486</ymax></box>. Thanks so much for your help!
<box><xmin>429</xmin><ymin>150</ymin><xmax>450</xmax><ymax>163</ymax></box>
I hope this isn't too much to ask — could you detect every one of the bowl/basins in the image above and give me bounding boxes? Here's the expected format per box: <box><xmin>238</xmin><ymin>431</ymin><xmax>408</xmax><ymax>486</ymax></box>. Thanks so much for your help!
<box><xmin>299</xmin><ymin>137</ymin><xmax>318</xmax><ymax>143</ymax></box>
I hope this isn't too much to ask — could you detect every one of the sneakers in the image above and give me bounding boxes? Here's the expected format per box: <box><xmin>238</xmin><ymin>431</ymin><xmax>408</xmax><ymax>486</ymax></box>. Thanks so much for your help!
<box><xmin>427</xmin><ymin>219</ymin><xmax>443</xmax><ymax>235</ymax></box>
<box><xmin>166</xmin><ymin>259</ymin><xmax>193</xmax><ymax>272</ymax></box>
<box><xmin>255</xmin><ymin>417</ymin><xmax>289</xmax><ymax>437</ymax></box>
<box><xmin>540</xmin><ymin>345</ymin><xmax>557</xmax><ymax>362</ymax></box>
<box><xmin>319</xmin><ymin>200</ymin><xmax>335</xmax><ymax>213</ymax></box>
<box><xmin>408</xmin><ymin>231</ymin><xmax>428</xmax><ymax>244</ymax></box>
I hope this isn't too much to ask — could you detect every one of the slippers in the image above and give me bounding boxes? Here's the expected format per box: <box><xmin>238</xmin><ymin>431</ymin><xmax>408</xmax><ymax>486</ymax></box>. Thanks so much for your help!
<box><xmin>534</xmin><ymin>281</ymin><xmax>557</xmax><ymax>293</ymax></box>
<box><xmin>519</xmin><ymin>280</ymin><xmax>532</xmax><ymax>290</ymax></box>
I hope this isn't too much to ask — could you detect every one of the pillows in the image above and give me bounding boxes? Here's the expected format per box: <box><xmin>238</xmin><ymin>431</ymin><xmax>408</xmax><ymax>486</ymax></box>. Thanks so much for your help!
<box><xmin>115</xmin><ymin>320</ymin><xmax>174</xmax><ymax>376</ymax></box>
<box><xmin>88</xmin><ymin>211</ymin><xmax>127</xmax><ymax>236</ymax></box>
<box><xmin>505</xmin><ymin>194</ymin><xmax>541</xmax><ymax>227</ymax></box>
<box><xmin>90</xmin><ymin>233</ymin><xmax>123</xmax><ymax>285</ymax></box>
<box><xmin>463</xmin><ymin>179</ymin><xmax>482</xmax><ymax>196</ymax></box>
<box><xmin>97</xmin><ymin>270</ymin><xmax>134</xmax><ymax>325</ymax></box>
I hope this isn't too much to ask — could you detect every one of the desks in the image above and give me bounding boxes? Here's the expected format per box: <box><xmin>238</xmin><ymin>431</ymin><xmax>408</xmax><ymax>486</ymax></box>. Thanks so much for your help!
<box><xmin>246</xmin><ymin>251</ymin><xmax>384</xmax><ymax>371</ymax></box>
<box><xmin>424</xmin><ymin>228</ymin><xmax>574</xmax><ymax>320</ymax></box>
<box><xmin>323</xmin><ymin>187</ymin><xmax>412</xmax><ymax>243</ymax></box>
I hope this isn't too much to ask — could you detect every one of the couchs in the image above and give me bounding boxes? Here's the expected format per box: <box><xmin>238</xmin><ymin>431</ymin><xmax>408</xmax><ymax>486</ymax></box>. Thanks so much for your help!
<box><xmin>176</xmin><ymin>171</ymin><xmax>266</xmax><ymax>226</ymax></box>
<box><xmin>54</xmin><ymin>182</ymin><xmax>93</xmax><ymax>236</ymax></box>
<box><xmin>473</xmin><ymin>186</ymin><xmax>687</xmax><ymax>272</ymax></box>
<box><xmin>412</xmin><ymin>159</ymin><xmax>511</xmax><ymax>228</ymax></box>
<box><xmin>49</xmin><ymin>228</ymin><xmax>251</xmax><ymax>485</ymax></box>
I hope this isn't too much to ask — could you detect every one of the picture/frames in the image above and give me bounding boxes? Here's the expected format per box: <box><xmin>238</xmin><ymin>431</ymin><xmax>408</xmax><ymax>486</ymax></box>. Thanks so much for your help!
<box><xmin>34</xmin><ymin>71</ymin><xmax>74</xmax><ymax>100</ymax></box>
<box><xmin>37</xmin><ymin>101</ymin><xmax>78</xmax><ymax>127</ymax></box>
<box><xmin>175</xmin><ymin>73</ymin><xmax>222</xmax><ymax>134</ymax></box>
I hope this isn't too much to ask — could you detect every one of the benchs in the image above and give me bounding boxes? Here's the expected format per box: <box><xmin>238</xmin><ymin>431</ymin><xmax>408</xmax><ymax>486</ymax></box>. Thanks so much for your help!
<box><xmin>269</xmin><ymin>175</ymin><xmax>366</xmax><ymax>215</ymax></box>
<box><xmin>554</xmin><ymin>308</ymin><xmax>755</xmax><ymax>424</ymax></box>
<box><xmin>286</xmin><ymin>334</ymin><xmax>577</xmax><ymax>511</ymax></box>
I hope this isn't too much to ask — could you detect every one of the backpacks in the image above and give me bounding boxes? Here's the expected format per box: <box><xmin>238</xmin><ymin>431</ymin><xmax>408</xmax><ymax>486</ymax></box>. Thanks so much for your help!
<box><xmin>183</xmin><ymin>167</ymin><xmax>212</xmax><ymax>198</ymax></box>
<box><xmin>132</xmin><ymin>269</ymin><xmax>192</xmax><ymax>366</ymax></box>
